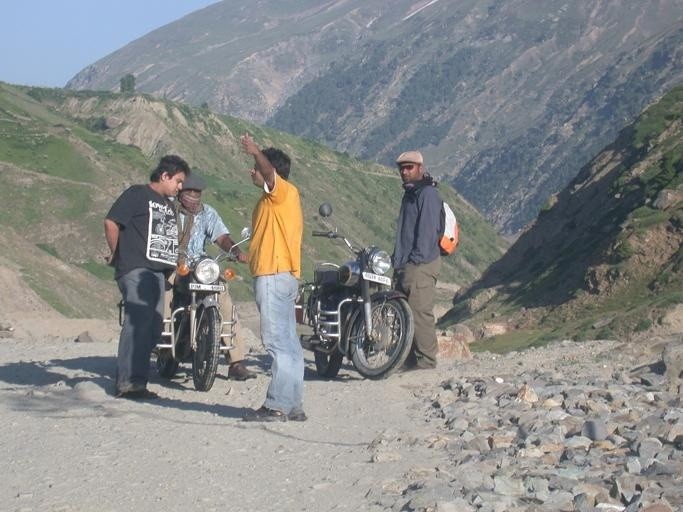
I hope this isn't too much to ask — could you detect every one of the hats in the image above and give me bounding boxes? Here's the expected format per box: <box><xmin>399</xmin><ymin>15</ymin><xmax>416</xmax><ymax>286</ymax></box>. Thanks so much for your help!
<box><xmin>182</xmin><ymin>173</ymin><xmax>205</xmax><ymax>190</ymax></box>
<box><xmin>396</xmin><ymin>152</ymin><xmax>423</xmax><ymax>164</ymax></box>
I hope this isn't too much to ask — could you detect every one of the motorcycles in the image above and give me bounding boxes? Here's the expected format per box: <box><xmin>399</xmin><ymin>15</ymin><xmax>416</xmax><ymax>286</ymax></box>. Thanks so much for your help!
<box><xmin>117</xmin><ymin>223</ymin><xmax>250</xmax><ymax>392</ymax></box>
<box><xmin>294</xmin><ymin>199</ymin><xmax>414</xmax><ymax>379</ymax></box>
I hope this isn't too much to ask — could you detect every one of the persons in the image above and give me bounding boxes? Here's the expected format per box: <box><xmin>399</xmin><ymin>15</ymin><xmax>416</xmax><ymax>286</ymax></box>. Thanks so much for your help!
<box><xmin>104</xmin><ymin>155</ymin><xmax>191</xmax><ymax>398</ymax></box>
<box><xmin>392</xmin><ymin>152</ymin><xmax>445</xmax><ymax>368</ymax></box>
<box><xmin>163</xmin><ymin>175</ymin><xmax>257</xmax><ymax>381</ymax></box>
<box><xmin>240</xmin><ymin>132</ymin><xmax>306</xmax><ymax>421</ymax></box>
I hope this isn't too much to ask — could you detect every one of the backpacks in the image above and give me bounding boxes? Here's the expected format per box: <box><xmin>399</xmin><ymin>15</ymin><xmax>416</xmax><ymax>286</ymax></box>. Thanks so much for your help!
<box><xmin>439</xmin><ymin>201</ymin><xmax>460</xmax><ymax>256</ymax></box>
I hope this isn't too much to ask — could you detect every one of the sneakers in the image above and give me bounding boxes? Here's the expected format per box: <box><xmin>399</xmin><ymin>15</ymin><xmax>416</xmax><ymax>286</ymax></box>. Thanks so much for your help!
<box><xmin>228</xmin><ymin>365</ymin><xmax>257</xmax><ymax>380</ymax></box>
<box><xmin>244</xmin><ymin>407</ymin><xmax>307</xmax><ymax>422</ymax></box>
<box><xmin>116</xmin><ymin>389</ymin><xmax>158</xmax><ymax>398</ymax></box>
<box><xmin>397</xmin><ymin>364</ymin><xmax>424</xmax><ymax>373</ymax></box>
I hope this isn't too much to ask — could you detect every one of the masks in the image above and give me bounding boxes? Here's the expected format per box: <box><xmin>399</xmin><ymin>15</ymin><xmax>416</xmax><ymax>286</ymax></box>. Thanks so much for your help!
<box><xmin>179</xmin><ymin>193</ymin><xmax>201</xmax><ymax>212</ymax></box>
